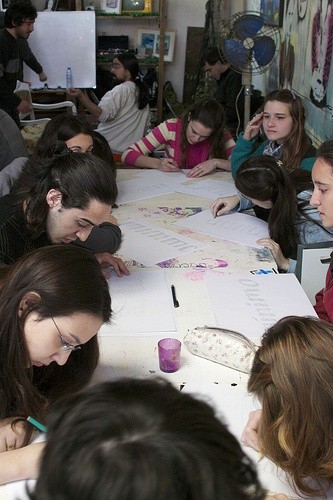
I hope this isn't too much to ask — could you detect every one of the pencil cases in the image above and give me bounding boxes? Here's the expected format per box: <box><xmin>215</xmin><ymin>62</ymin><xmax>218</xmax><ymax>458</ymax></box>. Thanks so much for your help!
<box><xmin>184</xmin><ymin>324</ymin><xmax>258</xmax><ymax>375</ymax></box>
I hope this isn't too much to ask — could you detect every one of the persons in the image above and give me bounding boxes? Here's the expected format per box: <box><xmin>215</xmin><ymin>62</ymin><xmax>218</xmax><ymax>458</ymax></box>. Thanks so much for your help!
<box><xmin>230</xmin><ymin>89</ymin><xmax>318</xmax><ymax>180</ymax></box>
<box><xmin>199</xmin><ymin>47</ymin><xmax>244</xmax><ymax>128</ymax></box>
<box><xmin>121</xmin><ymin>98</ymin><xmax>236</xmax><ymax>178</ymax></box>
<box><xmin>0</xmin><ymin>113</ymin><xmax>93</xmax><ymax>197</ymax></box>
<box><xmin>240</xmin><ymin>315</ymin><xmax>333</xmax><ymax>500</ymax></box>
<box><xmin>0</xmin><ymin>108</ymin><xmax>27</xmax><ymax>172</ymax></box>
<box><xmin>27</xmin><ymin>377</ymin><xmax>267</xmax><ymax>500</ymax></box>
<box><xmin>210</xmin><ymin>155</ymin><xmax>333</xmax><ymax>274</ymax></box>
<box><xmin>0</xmin><ymin>0</ymin><xmax>48</xmax><ymax>128</ymax></box>
<box><xmin>67</xmin><ymin>54</ymin><xmax>150</xmax><ymax>163</ymax></box>
<box><xmin>0</xmin><ymin>245</ymin><xmax>111</xmax><ymax>486</ymax></box>
<box><xmin>309</xmin><ymin>147</ymin><xmax>333</xmax><ymax>324</ymax></box>
<box><xmin>72</xmin><ymin>215</ymin><xmax>122</xmax><ymax>254</ymax></box>
<box><xmin>0</xmin><ymin>140</ymin><xmax>131</xmax><ymax>280</ymax></box>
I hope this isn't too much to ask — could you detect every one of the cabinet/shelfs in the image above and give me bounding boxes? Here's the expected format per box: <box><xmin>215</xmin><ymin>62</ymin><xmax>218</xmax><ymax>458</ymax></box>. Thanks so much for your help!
<box><xmin>76</xmin><ymin>0</ymin><xmax>163</xmax><ymax>123</ymax></box>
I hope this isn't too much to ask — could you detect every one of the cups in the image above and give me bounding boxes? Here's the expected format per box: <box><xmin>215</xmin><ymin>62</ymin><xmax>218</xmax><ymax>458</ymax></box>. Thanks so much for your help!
<box><xmin>157</xmin><ymin>338</ymin><xmax>181</xmax><ymax>373</ymax></box>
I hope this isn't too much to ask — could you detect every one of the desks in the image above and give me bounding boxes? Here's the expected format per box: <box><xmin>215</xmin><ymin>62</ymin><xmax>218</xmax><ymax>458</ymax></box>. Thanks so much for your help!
<box><xmin>0</xmin><ymin>168</ymin><xmax>319</xmax><ymax>500</ymax></box>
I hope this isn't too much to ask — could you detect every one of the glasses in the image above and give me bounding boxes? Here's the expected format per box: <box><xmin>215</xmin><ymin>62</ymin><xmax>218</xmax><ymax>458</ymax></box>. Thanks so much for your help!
<box><xmin>50</xmin><ymin>316</ymin><xmax>81</xmax><ymax>352</ymax></box>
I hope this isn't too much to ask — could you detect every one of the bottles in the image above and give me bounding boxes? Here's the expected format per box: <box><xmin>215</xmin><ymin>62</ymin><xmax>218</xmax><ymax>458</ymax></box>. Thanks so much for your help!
<box><xmin>66</xmin><ymin>68</ymin><xmax>72</xmax><ymax>88</ymax></box>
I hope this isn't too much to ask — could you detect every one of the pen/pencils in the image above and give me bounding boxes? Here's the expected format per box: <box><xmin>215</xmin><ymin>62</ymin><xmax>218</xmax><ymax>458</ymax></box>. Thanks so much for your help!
<box><xmin>257</xmin><ymin>453</ymin><xmax>267</xmax><ymax>461</ymax></box>
<box><xmin>27</xmin><ymin>416</ymin><xmax>48</xmax><ymax>434</ymax></box>
<box><xmin>170</xmin><ymin>285</ymin><xmax>179</xmax><ymax>307</ymax></box>
<box><xmin>213</xmin><ymin>206</ymin><xmax>224</xmax><ymax>219</ymax></box>
<box><xmin>164</xmin><ymin>152</ymin><xmax>184</xmax><ymax>174</ymax></box>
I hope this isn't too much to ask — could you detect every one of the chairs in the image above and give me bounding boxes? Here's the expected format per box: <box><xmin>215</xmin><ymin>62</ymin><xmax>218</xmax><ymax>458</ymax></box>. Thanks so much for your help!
<box><xmin>0</xmin><ymin>80</ymin><xmax>78</xmax><ymax>171</ymax></box>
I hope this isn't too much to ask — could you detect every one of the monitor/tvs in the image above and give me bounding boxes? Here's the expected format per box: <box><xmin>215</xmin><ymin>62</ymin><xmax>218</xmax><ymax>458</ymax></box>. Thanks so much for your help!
<box><xmin>122</xmin><ymin>0</ymin><xmax>152</xmax><ymax>14</ymax></box>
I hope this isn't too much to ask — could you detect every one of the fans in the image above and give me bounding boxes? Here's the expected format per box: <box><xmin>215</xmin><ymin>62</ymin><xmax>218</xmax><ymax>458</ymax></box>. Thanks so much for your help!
<box><xmin>216</xmin><ymin>10</ymin><xmax>282</xmax><ymax>137</ymax></box>
